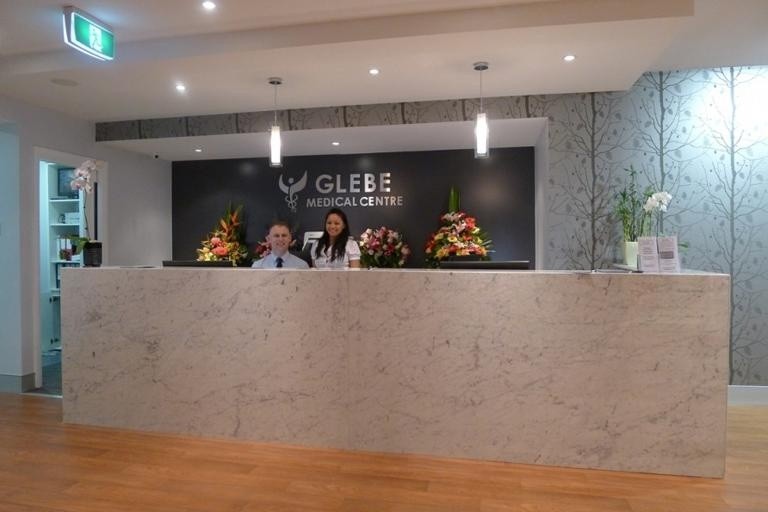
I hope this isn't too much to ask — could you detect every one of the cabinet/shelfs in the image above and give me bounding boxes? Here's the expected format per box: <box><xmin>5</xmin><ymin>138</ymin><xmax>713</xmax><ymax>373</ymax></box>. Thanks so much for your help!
<box><xmin>40</xmin><ymin>161</ymin><xmax>84</xmax><ymax>351</ymax></box>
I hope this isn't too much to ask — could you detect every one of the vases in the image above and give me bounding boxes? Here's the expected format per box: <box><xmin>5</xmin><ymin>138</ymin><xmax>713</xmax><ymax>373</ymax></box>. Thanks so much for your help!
<box><xmin>84</xmin><ymin>242</ymin><xmax>102</xmax><ymax>265</ymax></box>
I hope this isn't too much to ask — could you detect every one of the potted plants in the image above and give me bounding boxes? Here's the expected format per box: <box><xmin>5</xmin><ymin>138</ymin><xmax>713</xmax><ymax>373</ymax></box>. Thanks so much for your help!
<box><xmin>616</xmin><ymin>167</ymin><xmax>652</xmax><ymax>265</ymax></box>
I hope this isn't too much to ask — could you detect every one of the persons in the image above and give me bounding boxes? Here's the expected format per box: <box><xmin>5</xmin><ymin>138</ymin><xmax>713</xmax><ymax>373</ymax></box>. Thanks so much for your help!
<box><xmin>310</xmin><ymin>207</ymin><xmax>362</xmax><ymax>269</ymax></box>
<box><xmin>251</xmin><ymin>221</ymin><xmax>310</xmax><ymax>269</ymax></box>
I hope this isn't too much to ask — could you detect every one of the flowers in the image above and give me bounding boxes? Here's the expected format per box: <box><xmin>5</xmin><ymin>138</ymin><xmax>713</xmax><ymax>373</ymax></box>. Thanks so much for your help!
<box><xmin>197</xmin><ymin>200</ymin><xmax>248</xmax><ymax>266</ymax></box>
<box><xmin>358</xmin><ymin>226</ymin><xmax>412</xmax><ymax>269</ymax></box>
<box><xmin>70</xmin><ymin>160</ymin><xmax>106</xmax><ymax>254</ymax></box>
<box><xmin>425</xmin><ymin>188</ymin><xmax>495</xmax><ymax>267</ymax></box>
<box><xmin>642</xmin><ymin>190</ymin><xmax>670</xmax><ymax>236</ymax></box>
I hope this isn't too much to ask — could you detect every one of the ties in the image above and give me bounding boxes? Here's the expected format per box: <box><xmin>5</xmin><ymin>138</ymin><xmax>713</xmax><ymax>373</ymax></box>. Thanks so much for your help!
<box><xmin>276</xmin><ymin>257</ymin><xmax>283</xmax><ymax>267</ymax></box>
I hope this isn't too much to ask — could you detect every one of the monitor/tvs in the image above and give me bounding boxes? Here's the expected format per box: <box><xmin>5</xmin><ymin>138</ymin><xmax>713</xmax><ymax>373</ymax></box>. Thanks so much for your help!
<box><xmin>440</xmin><ymin>260</ymin><xmax>531</xmax><ymax>269</ymax></box>
<box><xmin>162</xmin><ymin>260</ymin><xmax>233</xmax><ymax>267</ymax></box>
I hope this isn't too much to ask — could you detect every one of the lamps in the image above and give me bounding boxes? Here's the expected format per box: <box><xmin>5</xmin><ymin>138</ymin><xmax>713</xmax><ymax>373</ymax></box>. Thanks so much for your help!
<box><xmin>268</xmin><ymin>79</ymin><xmax>283</xmax><ymax>168</ymax></box>
<box><xmin>473</xmin><ymin>63</ymin><xmax>490</xmax><ymax>158</ymax></box>
